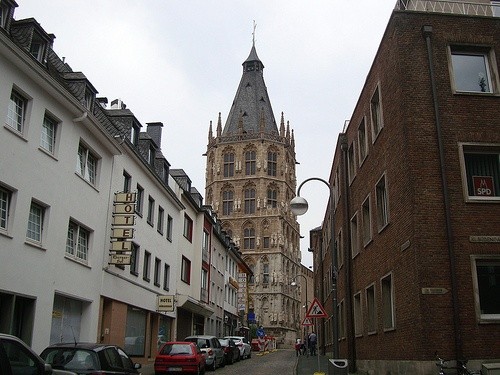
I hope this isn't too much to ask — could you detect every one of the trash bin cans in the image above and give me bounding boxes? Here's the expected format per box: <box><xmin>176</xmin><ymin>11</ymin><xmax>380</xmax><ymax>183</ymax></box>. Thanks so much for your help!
<box><xmin>328</xmin><ymin>358</ymin><xmax>349</xmax><ymax>375</ymax></box>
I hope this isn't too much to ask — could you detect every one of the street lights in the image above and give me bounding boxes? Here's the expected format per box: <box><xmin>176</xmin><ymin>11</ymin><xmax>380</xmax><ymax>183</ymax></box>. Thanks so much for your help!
<box><xmin>288</xmin><ymin>176</ymin><xmax>340</xmax><ymax>361</ymax></box>
<box><xmin>290</xmin><ymin>274</ymin><xmax>311</xmax><ymax>360</ymax></box>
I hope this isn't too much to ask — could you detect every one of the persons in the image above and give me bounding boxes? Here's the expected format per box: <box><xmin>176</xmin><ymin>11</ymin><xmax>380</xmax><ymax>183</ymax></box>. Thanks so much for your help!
<box><xmin>295</xmin><ymin>338</ymin><xmax>302</xmax><ymax>357</ymax></box>
<box><xmin>309</xmin><ymin>331</ymin><xmax>317</xmax><ymax>356</ymax></box>
<box><xmin>303</xmin><ymin>336</ymin><xmax>308</xmax><ymax>355</ymax></box>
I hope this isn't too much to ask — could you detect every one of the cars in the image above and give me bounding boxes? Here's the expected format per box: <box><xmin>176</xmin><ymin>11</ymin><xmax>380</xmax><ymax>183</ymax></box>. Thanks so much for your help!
<box><xmin>153</xmin><ymin>341</ymin><xmax>206</xmax><ymax>375</ymax></box>
<box><xmin>183</xmin><ymin>335</ymin><xmax>224</xmax><ymax>368</ymax></box>
<box><xmin>0</xmin><ymin>333</ymin><xmax>78</xmax><ymax>375</ymax></box>
<box><xmin>224</xmin><ymin>335</ymin><xmax>253</xmax><ymax>359</ymax></box>
<box><xmin>37</xmin><ymin>341</ymin><xmax>142</xmax><ymax>375</ymax></box>
<box><xmin>216</xmin><ymin>338</ymin><xmax>240</xmax><ymax>365</ymax></box>
